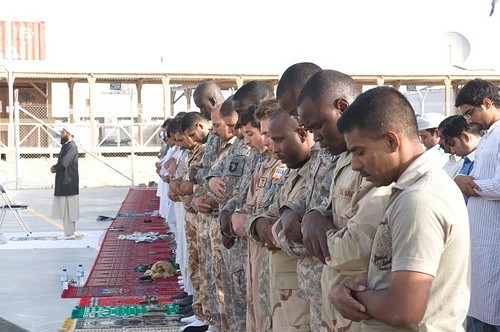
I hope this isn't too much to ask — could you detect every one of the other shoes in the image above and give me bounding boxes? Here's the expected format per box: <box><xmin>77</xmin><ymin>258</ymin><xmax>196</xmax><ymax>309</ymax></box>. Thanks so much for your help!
<box><xmin>57</xmin><ymin>234</ymin><xmax>75</xmax><ymax>240</ymax></box>
<box><xmin>146</xmin><ymin>303</ymin><xmax>168</xmax><ymax>312</ymax></box>
<box><xmin>139</xmin><ymin>294</ymin><xmax>156</xmax><ymax>303</ymax></box>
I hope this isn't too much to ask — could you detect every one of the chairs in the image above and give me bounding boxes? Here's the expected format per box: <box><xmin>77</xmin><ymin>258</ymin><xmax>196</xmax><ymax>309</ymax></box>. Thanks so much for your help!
<box><xmin>0</xmin><ymin>184</ymin><xmax>33</xmax><ymax>237</ymax></box>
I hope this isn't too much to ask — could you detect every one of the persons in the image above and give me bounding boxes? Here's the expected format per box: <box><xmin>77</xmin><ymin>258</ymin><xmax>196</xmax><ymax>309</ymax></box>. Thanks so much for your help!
<box><xmin>50</xmin><ymin>124</ymin><xmax>81</xmax><ymax>240</ymax></box>
<box><xmin>155</xmin><ymin>62</ymin><xmax>500</xmax><ymax>332</ymax></box>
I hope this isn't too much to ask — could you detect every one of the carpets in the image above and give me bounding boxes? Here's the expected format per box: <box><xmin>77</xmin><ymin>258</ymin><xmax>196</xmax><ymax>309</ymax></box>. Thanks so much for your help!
<box><xmin>58</xmin><ymin>189</ymin><xmax>185</xmax><ymax>332</ymax></box>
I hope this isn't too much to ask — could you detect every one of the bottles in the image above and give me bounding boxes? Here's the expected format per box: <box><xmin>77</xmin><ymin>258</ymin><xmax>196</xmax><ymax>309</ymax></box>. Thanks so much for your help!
<box><xmin>61</xmin><ymin>269</ymin><xmax>68</xmax><ymax>290</ymax></box>
<box><xmin>77</xmin><ymin>265</ymin><xmax>84</xmax><ymax>286</ymax></box>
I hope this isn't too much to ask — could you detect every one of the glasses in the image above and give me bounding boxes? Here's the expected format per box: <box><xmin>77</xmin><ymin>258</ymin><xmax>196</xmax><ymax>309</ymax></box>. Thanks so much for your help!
<box><xmin>463</xmin><ymin>105</ymin><xmax>476</xmax><ymax>120</ymax></box>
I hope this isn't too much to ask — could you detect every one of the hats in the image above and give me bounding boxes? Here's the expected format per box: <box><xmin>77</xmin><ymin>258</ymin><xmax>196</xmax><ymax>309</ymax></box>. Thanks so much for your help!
<box><xmin>63</xmin><ymin>125</ymin><xmax>76</xmax><ymax>135</ymax></box>
<box><xmin>417</xmin><ymin>112</ymin><xmax>446</xmax><ymax>130</ymax></box>
